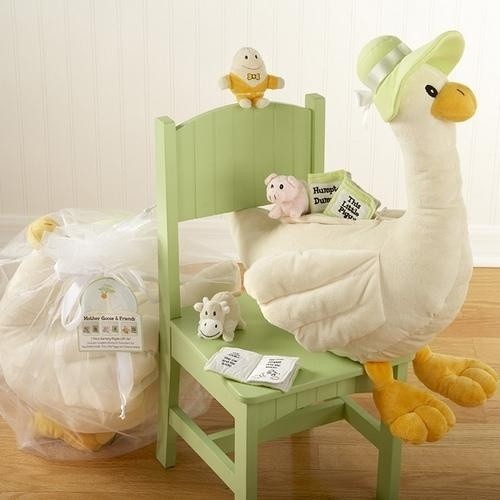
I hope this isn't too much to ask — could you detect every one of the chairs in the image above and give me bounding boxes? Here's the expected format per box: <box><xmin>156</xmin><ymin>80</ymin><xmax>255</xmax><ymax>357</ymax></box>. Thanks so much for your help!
<box><xmin>152</xmin><ymin>94</ymin><xmax>417</xmax><ymax>500</ymax></box>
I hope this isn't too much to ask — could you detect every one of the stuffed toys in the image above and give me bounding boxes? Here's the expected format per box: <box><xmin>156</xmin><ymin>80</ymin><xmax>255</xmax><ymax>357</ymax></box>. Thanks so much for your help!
<box><xmin>218</xmin><ymin>44</ymin><xmax>287</xmax><ymax>110</ymax></box>
<box><xmin>1</xmin><ymin>214</ymin><xmax>242</xmax><ymax>454</ymax></box>
<box><xmin>263</xmin><ymin>171</ymin><xmax>310</xmax><ymax>220</ymax></box>
<box><xmin>239</xmin><ymin>30</ymin><xmax>500</xmax><ymax>446</ymax></box>
<box><xmin>193</xmin><ymin>291</ymin><xmax>247</xmax><ymax>344</ymax></box>
